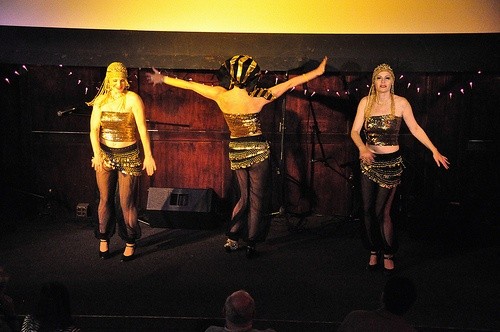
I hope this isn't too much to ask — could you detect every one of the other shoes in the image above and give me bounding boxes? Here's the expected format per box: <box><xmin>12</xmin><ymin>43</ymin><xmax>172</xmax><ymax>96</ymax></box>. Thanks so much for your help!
<box><xmin>225</xmin><ymin>243</ymin><xmax>243</xmax><ymax>254</ymax></box>
<box><xmin>246</xmin><ymin>244</ymin><xmax>258</xmax><ymax>259</ymax></box>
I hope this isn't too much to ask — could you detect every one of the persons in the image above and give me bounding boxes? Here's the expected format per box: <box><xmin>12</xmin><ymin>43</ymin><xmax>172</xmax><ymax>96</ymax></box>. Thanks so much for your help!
<box><xmin>205</xmin><ymin>290</ymin><xmax>276</xmax><ymax>332</ymax></box>
<box><xmin>337</xmin><ymin>276</ymin><xmax>418</xmax><ymax>332</ymax></box>
<box><xmin>0</xmin><ymin>269</ymin><xmax>82</xmax><ymax>332</ymax></box>
<box><xmin>85</xmin><ymin>62</ymin><xmax>156</xmax><ymax>262</ymax></box>
<box><xmin>146</xmin><ymin>54</ymin><xmax>328</xmax><ymax>258</ymax></box>
<box><xmin>350</xmin><ymin>63</ymin><xmax>450</xmax><ymax>275</ymax></box>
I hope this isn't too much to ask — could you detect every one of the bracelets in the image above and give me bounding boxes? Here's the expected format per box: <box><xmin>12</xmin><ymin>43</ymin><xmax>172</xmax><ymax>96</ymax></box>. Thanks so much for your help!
<box><xmin>303</xmin><ymin>74</ymin><xmax>309</xmax><ymax>82</ymax></box>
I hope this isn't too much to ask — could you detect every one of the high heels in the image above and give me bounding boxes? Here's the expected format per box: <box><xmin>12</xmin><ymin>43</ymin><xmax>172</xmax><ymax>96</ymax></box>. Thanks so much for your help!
<box><xmin>368</xmin><ymin>252</ymin><xmax>378</xmax><ymax>272</ymax></box>
<box><xmin>120</xmin><ymin>243</ymin><xmax>135</xmax><ymax>263</ymax></box>
<box><xmin>99</xmin><ymin>240</ymin><xmax>109</xmax><ymax>260</ymax></box>
<box><xmin>383</xmin><ymin>256</ymin><xmax>395</xmax><ymax>276</ymax></box>
